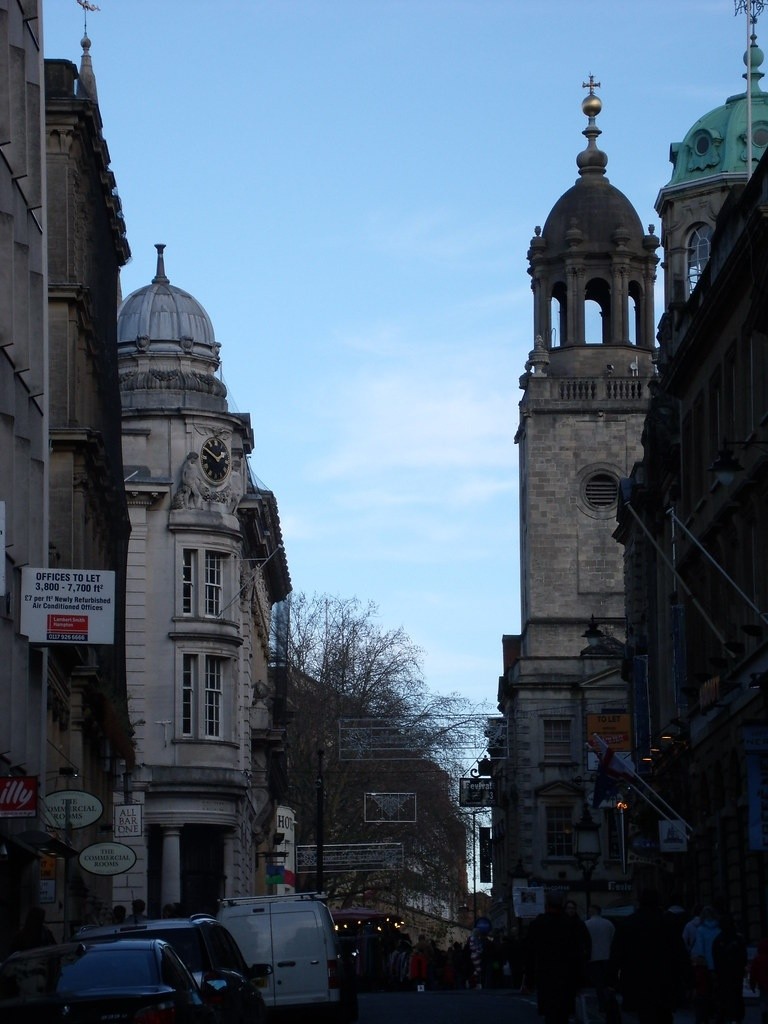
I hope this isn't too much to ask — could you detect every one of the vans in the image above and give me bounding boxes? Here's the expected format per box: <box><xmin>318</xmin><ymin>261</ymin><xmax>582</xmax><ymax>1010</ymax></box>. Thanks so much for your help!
<box><xmin>215</xmin><ymin>890</ymin><xmax>358</xmax><ymax>1024</ymax></box>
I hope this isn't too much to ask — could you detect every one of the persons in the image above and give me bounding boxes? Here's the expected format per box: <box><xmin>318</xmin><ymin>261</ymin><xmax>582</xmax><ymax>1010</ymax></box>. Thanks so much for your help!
<box><xmin>10</xmin><ymin>899</ymin><xmax>748</xmax><ymax>1024</ymax></box>
<box><xmin>749</xmin><ymin>936</ymin><xmax>768</xmax><ymax>1024</ymax></box>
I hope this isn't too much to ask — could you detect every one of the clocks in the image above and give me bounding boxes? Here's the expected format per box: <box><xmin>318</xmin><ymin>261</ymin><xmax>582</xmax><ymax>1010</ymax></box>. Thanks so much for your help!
<box><xmin>201</xmin><ymin>437</ymin><xmax>231</xmax><ymax>481</ymax></box>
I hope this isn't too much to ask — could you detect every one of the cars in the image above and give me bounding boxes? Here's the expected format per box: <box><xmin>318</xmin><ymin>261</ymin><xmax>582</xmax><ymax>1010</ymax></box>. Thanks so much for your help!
<box><xmin>1</xmin><ymin>937</ymin><xmax>230</xmax><ymax>1024</ymax></box>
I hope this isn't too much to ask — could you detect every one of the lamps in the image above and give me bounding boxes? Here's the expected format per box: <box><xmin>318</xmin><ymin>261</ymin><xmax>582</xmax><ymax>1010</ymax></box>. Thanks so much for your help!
<box><xmin>580</xmin><ymin>614</ymin><xmax>629</xmax><ymax>660</ymax></box>
<box><xmin>570</xmin><ymin>777</ymin><xmax>606</xmax><ymax>875</ymax></box>
<box><xmin>642</xmin><ymin>672</ymin><xmax>767</xmax><ymax>761</ymax></box>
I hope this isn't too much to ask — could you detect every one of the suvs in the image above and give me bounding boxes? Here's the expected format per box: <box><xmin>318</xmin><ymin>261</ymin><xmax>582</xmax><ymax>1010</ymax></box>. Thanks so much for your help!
<box><xmin>72</xmin><ymin>910</ymin><xmax>274</xmax><ymax>1024</ymax></box>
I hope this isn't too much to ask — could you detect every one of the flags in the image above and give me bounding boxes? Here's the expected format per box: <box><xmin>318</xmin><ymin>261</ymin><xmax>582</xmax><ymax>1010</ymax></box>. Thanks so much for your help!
<box><xmin>590</xmin><ymin>739</ymin><xmax>632</xmax><ymax>808</ymax></box>
